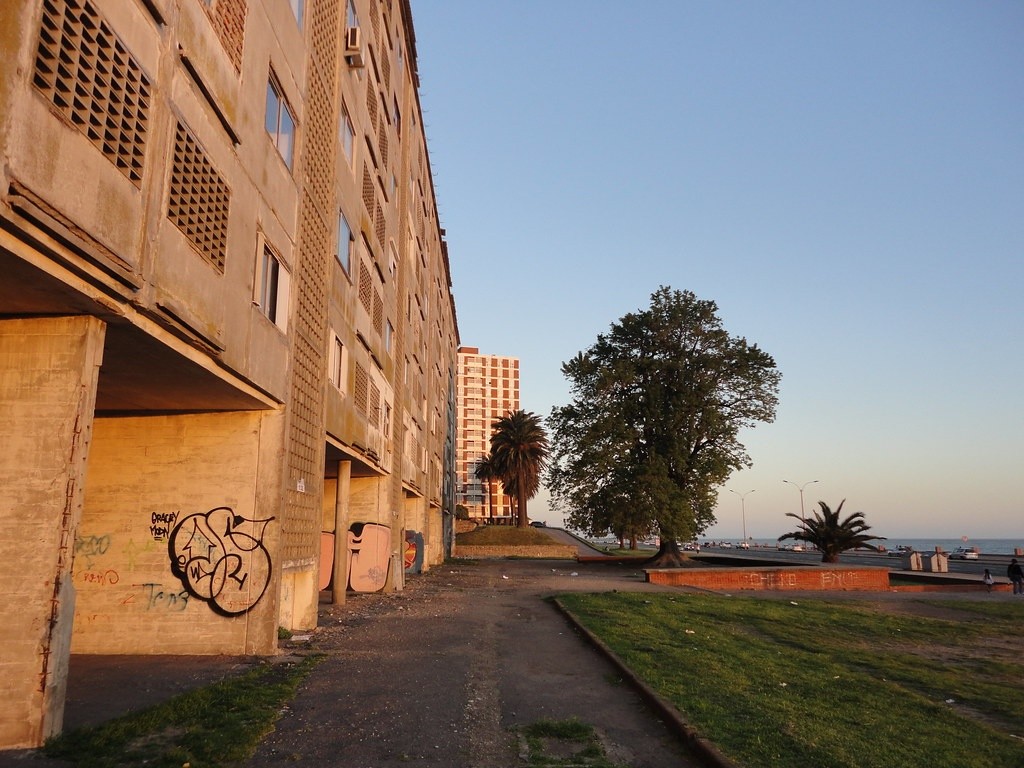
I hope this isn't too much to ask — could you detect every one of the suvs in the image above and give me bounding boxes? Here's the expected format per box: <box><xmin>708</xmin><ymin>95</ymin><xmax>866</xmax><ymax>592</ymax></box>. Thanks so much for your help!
<box><xmin>530</xmin><ymin>522</ymin><xmax>543</xmax><ymax>528</ymax></box>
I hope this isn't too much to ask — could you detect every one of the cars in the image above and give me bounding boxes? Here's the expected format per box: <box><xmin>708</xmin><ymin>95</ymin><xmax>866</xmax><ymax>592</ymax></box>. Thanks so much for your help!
<box><xmin>887</xmin><ymin>547</ymin><xmax>908</xmax><ymax>557</ymax></box>
<box><xmin>791</xmin><ymin>545</ymin><xmax>802</xmax><ymax>552</ymax></box>
<box><xmin>720</xmin><ymin>543</ymin><xmax>732</xmax><ymax>549</ymax></box>
<box><xmin>949</xmin><ymin>548</ymin><xmax>978</xmax><ymax>559</ymax></box>
<box><xmin>644</xmin><ymin>541</ymin><xmax>650</xmax><ymax>545</ymax></box>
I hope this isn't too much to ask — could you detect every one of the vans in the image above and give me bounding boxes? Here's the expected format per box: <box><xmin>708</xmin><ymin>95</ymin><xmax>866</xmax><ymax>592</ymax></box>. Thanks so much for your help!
<box><xmin>677</xmin><ymin>543</ymin><xmax>683</xmax><ymax>550</ymax></box>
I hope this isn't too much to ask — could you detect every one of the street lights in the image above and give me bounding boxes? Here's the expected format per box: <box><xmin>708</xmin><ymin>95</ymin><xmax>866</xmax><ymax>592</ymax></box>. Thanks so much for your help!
<box><xmin>781</xmin><ymin>479</ymin><xmax>820</xmax><ymax>553</ymax></box>
<box><xmin>728</xmin><ymin>488</ymin><xmax>756</xmax><ymax>550</ymax></box>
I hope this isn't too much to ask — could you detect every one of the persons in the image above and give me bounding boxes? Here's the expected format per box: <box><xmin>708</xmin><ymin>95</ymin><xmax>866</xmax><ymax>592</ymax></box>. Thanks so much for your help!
<box><xmin>1007</xmin><ymin>559</ymin><xmax>1024</xmax><ymax>595</ymax></box>
<box><xmin>891</xmin><ymin>544</ymin><xmax>904</xmax><ymax>557</ymax></box>
<box><xmin>983</xmin><ymin>569</ymin><xmax>994</xmax><ymax>594</ymax></box>
<box><xmin>543</xmin><ymin>521</ymin><xmax>546</xmax><ymax>527</ymax></box>
<box><xmin>696</xmin><ymin>543</ymin><xmax>700</xmax><ymax>553</ymax></box>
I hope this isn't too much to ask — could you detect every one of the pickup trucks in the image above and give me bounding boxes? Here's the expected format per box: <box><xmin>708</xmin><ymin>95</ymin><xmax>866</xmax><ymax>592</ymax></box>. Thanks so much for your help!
<box><xmin>736</xmin><ymin>541</ymin><xmax>750</xmax><ymax>549</ymax></box>
<box><xmin>684</xmin><ymin>545</ymin><xmax>694</xmax><ymax>550</ymax></box>
